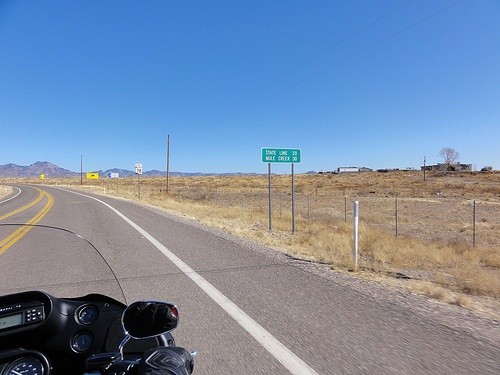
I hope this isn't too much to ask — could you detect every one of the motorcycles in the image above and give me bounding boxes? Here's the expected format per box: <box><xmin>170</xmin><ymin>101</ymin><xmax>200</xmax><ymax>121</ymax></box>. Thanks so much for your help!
<box><xmin>0</xmin><ymin>223</ymin><xmax>195</xmax><ymax>375</ymax></box>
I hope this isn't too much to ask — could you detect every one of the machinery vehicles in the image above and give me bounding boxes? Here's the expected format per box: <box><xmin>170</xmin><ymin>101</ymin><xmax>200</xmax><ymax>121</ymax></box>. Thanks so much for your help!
<box><xmin>334</xmin><ymin>167</ymin><xmax>358</xmax><ymax>174</ymax></box>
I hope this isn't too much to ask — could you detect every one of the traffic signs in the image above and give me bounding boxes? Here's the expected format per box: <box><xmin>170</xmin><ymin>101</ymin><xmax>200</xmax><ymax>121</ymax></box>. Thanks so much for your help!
<box><xmin>134</xmin><ymin>163</ymin><xmax>142</xmax><ymax>174</ymax></box>
<box><xmin>260</xmin><ymin>147</ymin><xmax>301</xmax><ymax>164</ymax></box>
